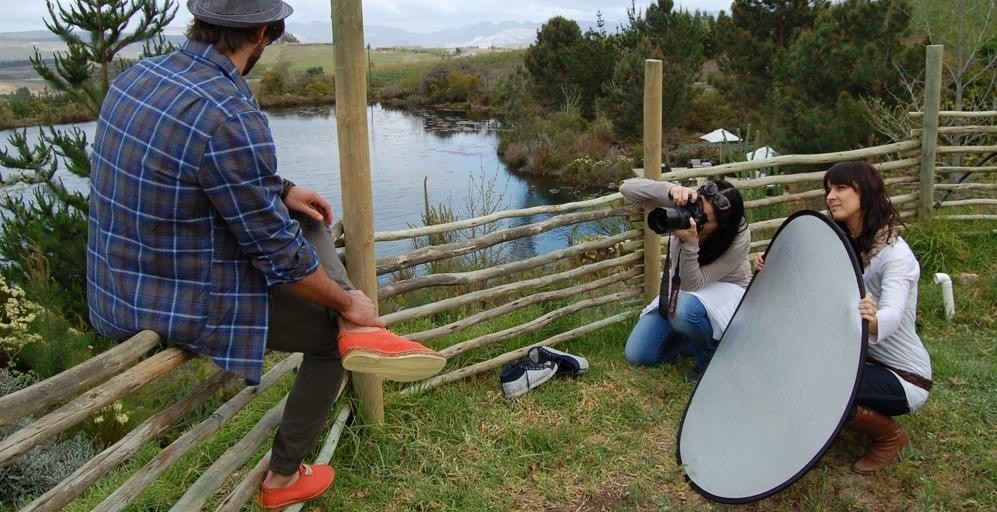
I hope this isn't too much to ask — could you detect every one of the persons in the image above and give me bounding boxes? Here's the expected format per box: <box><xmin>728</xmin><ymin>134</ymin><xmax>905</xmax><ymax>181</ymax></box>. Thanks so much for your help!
<box><xmin>754</xmin><ymin>162</ymin><xmax>933</xmax><ymax>474</ymax></box>
<box><xmin>86</xmin><ymin>0</ymin><xmax>447</xmax><ymax>510</ymax></box>
<box><xmin>618</xmin><ymin>178</ymin><xmax>752</xmax><ymax>382</ymax></box>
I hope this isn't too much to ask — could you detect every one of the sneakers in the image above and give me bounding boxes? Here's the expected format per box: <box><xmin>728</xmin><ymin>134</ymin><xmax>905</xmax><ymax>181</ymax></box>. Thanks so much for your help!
<box><xmin>528</xmin><ymin>345</ymin><xmax>588</xmax><ymax>375</ymax></box>
<box><xmin>336</xmin><ymin>327</ymin><xmax>447</xmax><ymax>382</ymax></box>
<box><xmin>499</xmin><ymin>361</ymin><xmax>558</xmax><ymax>398</ymax></box>
<box><xmin>256</xmin><ymin>464</ymin><xmax>334</xmax><ymax>511</ymax></box>
<box><xmin>687</xmin><ymin>365</ymin><xmax>706</xmax><ymax>383</ymax></box>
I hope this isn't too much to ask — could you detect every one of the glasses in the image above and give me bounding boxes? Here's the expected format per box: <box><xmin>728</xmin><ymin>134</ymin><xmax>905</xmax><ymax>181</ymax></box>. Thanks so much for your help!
<box><xmin>697</xmin><ymin>181</ymin><xmax>731</xmax><ymax>211</ymax></box>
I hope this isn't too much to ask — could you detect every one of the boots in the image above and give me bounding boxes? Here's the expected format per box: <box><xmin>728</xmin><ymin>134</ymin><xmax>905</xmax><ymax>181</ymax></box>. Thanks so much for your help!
<box><xmin>844</xmin><ymin>405</ymin><xmax>913</xmax><ymax>475</ymax></box>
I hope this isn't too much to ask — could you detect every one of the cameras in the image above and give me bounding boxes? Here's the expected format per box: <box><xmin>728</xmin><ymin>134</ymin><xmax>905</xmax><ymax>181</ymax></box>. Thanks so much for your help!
<box><xmin>647</xmin><ymin>197</ymin><xmax>709</xmax><ymax>235</ymax></box>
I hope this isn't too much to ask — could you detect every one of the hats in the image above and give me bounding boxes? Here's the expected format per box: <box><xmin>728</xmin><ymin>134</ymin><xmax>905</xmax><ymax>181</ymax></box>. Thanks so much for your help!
<box><xmin>186</xmin><ymin>0</ymin><xmax>294</xmax><ymax>28</ymax></box>
